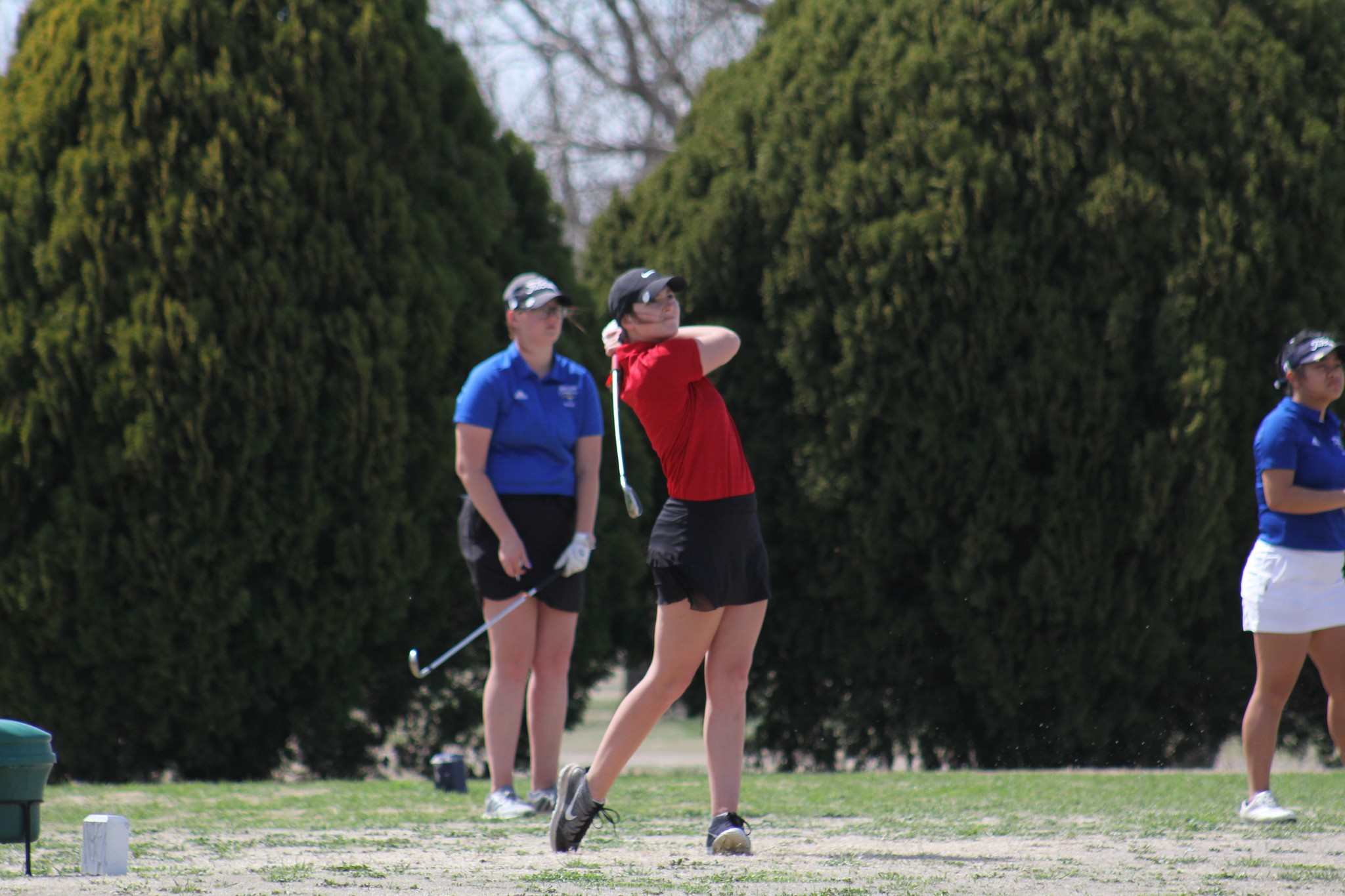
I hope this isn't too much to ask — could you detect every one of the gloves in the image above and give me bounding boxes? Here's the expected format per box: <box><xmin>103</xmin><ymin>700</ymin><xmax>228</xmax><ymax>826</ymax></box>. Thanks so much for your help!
<box><xmin>554</xmin><ymin>531</ymin><xmax>594</xmax><ymax>578</ymax></box>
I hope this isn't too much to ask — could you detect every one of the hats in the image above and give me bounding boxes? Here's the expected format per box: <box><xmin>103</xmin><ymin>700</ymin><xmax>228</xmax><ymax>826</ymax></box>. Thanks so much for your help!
<box><xmin>609</xmin><ymin>268</ymin><xmax>686</xmax><ymax>320</ymax></box>
<box><xmin>1280</xmin><ymin>329</ymin><xmax>1345</xmax><ymax>374</ymax></box>
<box><xmin>502</xmin><ymin>272</ymin><xmax>573</xmax><ymax>310</ymax></box>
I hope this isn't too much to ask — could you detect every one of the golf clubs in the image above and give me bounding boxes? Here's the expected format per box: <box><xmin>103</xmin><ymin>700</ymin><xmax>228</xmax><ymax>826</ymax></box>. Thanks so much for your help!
<box><xmin>404</xmin><ymin>559</ymin><xmax>565</xmax><ymax>681</ymax></box>
<box><xmin>604</xmin><ymin>332</ymin><xmax>641</xmax><ymax>525</ymax></box>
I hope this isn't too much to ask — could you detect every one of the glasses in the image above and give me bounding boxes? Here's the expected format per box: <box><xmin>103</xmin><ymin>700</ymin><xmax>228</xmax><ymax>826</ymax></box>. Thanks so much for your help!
<box><xmin>530</xmin><ymin>304</ymin><xmax>568</xmax><ymax>320</ymax></box>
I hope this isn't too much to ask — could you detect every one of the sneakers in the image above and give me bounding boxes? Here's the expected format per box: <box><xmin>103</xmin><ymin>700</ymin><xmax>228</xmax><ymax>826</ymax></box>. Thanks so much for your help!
<box><xmin>705</xmin><ymin>812</ymin><xmax>752</xmax><ymax>855</ymax></box>
<box><xmin>525</xmin><ymin>786</ymin><xmax>557</xmax><ymax>812</ymax></box>
<box><xmin>1238</xmin><ymin>790</ymin><xmax>1297</xmax><ymax>825</ymax></box>
<box><xmin>481</xmin><ymin>784</ymin><xmax>536</xmax><ymax>820</ymax></box>
<box><xmin>549</xmin><ymin>764</ymin><xmax>619</xmax><ymax>853</ymax></box>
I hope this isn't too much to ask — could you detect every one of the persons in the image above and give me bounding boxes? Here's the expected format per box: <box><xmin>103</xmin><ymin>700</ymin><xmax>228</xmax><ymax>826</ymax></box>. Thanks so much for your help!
<box><xmin>452</xmin><ymin>271</ymin><xmax>605</xmax><ymax>820</ymax></box>
<box><xmin>1239</xmin><ymin>329</ymin><xmax>1345</xmax><ymax>822</ymax></box>
<box><xmin>550</xmin><ymin>267</ymin><xmax>774</xmax><ymax>857</ymax></box>
